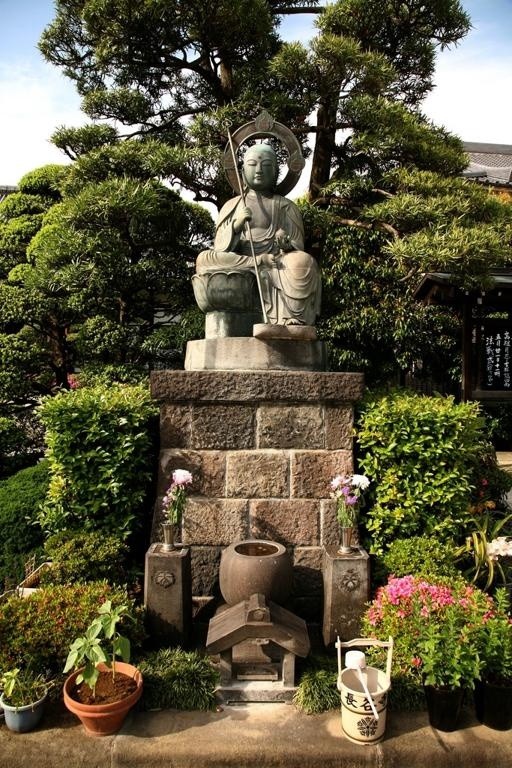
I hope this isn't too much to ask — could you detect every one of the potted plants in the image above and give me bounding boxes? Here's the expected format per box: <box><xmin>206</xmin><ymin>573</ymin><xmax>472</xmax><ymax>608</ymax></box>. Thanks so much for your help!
<box><xmin>63</xmin><ymin>599</ymin><xmax>143</xmax><ymax>736</ymax></box>
<box><xmin>0</xmin><ymin>656</ymin><xmax>49</xmax><ymax>732</ymax></box>
<box><xmin>15</xmin><ymin>530</ymin><xmax>131</xmax><ymax>607</ymax></box>
<box><xmin>417</xmin><ymin>585</ymin><xmax>512</xmax><ymax>732</ymax></box>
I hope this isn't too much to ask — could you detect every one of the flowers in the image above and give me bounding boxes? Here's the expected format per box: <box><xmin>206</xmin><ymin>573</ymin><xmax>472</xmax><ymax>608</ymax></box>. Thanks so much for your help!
<box><xmin>453</xmin><ymin>506</ymin><xmax>512</xmax><ymax>584</ymax></box>
<box><xmin>328</xmin><ymin>473</ymin><xmax>371</xmax><ymax>528</ymax></box>
<box><xmin>161</xmin><ymin>469</ymin><xmax>193</xmax><ymax>525</ymax></box>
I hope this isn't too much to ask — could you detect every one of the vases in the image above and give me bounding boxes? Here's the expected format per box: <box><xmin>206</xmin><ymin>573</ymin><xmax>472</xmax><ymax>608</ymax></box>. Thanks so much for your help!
<box><xmin>161</xmin><ymin>520</ymin><xmax>175</xmax><ymax>551</ymax></box>
<box><xmin>339</xmin><ymin>524</ymin><xmax>354</xmax><ymax>554</ymax></box>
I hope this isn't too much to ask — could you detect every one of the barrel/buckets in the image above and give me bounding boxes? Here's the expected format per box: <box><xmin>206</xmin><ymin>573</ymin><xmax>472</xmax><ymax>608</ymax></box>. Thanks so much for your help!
<box><xmin>334</xmin><ymin>636</ymin><xmax>394</xmax><ymax>747</ymax></box>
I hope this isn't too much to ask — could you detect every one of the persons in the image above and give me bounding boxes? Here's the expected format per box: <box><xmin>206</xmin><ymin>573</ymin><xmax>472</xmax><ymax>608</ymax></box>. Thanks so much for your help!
<box><xmin>194</xmin><ymin>143</ymin><xmax>322</xmax><ymax>325</ymax></box>
